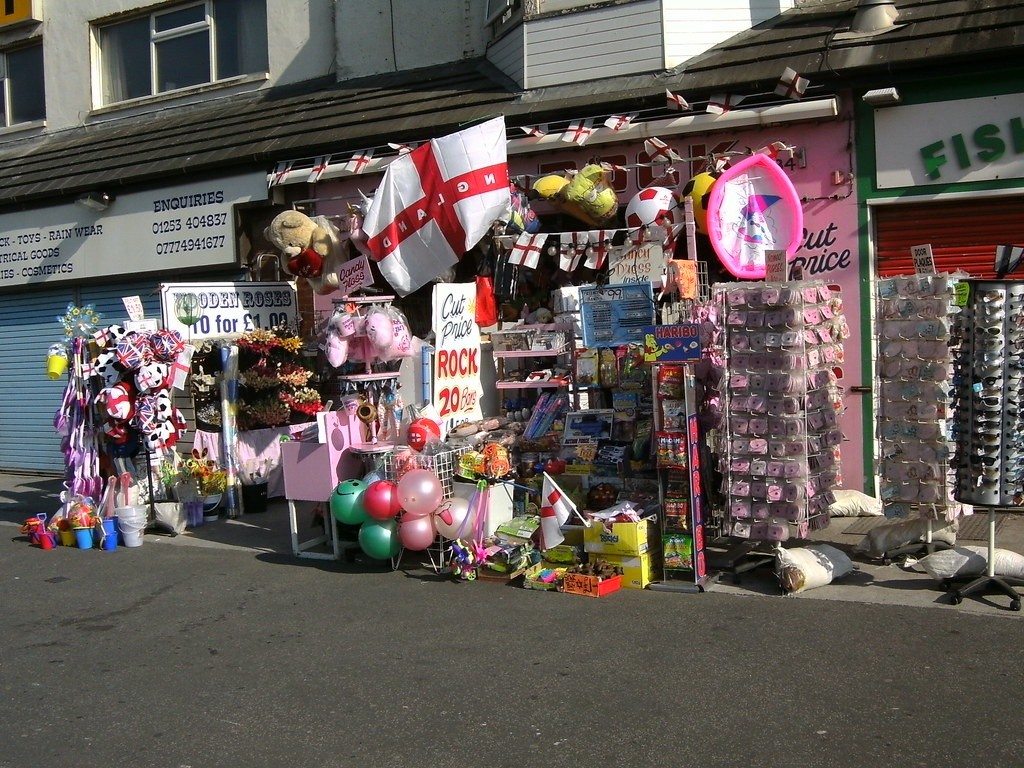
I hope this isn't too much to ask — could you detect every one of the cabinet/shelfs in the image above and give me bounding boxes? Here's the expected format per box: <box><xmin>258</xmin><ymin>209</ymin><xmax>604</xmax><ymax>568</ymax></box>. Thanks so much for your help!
<box><xmin>648</xmin><ymin>364</ymin><xmax>725</xmax><ymax>592</ymax></box>
<box><xmin>489</xmin><ymin>318</ymin><xmax>579</xmax><ymax>417</ymax></box>
<box><xmin>332</xmin><ymin>295</ymin><xmax>400</xmax><ymax>473</ymax></box>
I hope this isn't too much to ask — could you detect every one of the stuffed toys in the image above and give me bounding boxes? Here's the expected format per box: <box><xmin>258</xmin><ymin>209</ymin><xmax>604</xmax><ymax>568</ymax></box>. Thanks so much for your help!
<box><xmin>343</xmin><ymin>188</ymin><xmax>378</xmax><ymax>261</ymax></box>
<box><xmin>264</xmin><ymin>210</ymin><xmax>342</xmax><ymax>295</ymax></box>
<box><xmin>525</xmin><ymin>163</ymin><xmax>620</xmax><ymax>227</ymax></box>
<box><xmin>536</xmin><ymin>308</ymin><xmax>552</xmax><ymax>323</ymax></box>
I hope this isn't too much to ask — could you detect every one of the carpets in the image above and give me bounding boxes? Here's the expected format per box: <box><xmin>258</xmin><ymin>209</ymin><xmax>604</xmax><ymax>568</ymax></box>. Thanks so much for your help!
<box><xmin>844</xmin><ymin>512</ymin><xmax>1007</xmax><ymax>540</ymax></box>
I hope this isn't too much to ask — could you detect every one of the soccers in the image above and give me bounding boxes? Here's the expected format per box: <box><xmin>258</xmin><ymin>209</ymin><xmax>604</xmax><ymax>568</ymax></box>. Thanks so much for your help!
<box><xmin>680</xmin><ymin>172</ymin><xmax>723</xmax><ymax>235</ymax></box>
<box><xmin>624</xmin><ymin>186</ymin><xmax>685</xmax><ymax>245</ymax></box>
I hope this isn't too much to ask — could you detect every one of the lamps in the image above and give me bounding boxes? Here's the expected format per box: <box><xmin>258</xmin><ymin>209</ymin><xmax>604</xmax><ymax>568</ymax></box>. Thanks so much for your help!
<box><xmin>74</xmin><ymin>191</ymin><xmax>117</xmax><ymax>213</ymax></box>
<box><xmin>863</xmin><ymin>88</ymin><xmax>903</xmax><ymax>109</ymax></box>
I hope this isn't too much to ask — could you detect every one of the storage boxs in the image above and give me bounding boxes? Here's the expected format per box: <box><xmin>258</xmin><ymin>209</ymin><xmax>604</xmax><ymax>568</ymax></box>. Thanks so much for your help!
<box><xmin>383</xmin><ymin>445</ymin><xmax>474</xmax><ymax>575</ymax></box>
<box><xmin>526</xmin><ymin>568</ymin><xmax>568</xmax><ymax>591</ymax></box>
<box><xmin>616</xmin><ymin>491</ymin><xmax>660</xmax><ymax>516</ymax></box>
<box><xmin>625</xmin><ymin>478</ymin><xmax>658</xmax><ymax>492</ymax></box>
<box><xmin>489</xmin><ymin>327</ymin><xmax>652</xmax><ymax>475</ymax></box>
<box><xmin>598</xmin><ymin>575</ymin><xmax>621</xmax><ymax>595</ymax></box>
<box><xmin>543</xmin><ymin>529</ymin><xmax>590</xmax><ymax>564</ymax></box>
<box><xmin>551</xmin><ymin>286</ymin><xmax>581</xmax><ymax>313</ymax></box>
<box><xmin>553</xmin><ymin>313</ymin><xmax>583</xmax><ymax>338</ymax></box>
<box><xmin>588</xmin><ymin>545</ymin><xmax>662</xmax><ymax>589</ymax></box>
<box><xmin>449</xmin><ymin>479</ymin><xmax>516</xmax><ymax>544</ymax></box>
<box><xmin>583</xmin><ymin>513</ymin><xmax>660</xmax><ymax>557</ymax></box>
<box><xmin>574</xmin><ymin>339</ymin><xmax>585</xmax><ymax>348</ymax></box>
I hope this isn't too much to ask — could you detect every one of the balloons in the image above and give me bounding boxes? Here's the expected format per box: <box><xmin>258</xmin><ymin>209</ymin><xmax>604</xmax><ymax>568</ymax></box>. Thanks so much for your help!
<box><xmin>330</xmin><ymin>469</ymin><xmax>476</xmax><ymax>559</ymax></box>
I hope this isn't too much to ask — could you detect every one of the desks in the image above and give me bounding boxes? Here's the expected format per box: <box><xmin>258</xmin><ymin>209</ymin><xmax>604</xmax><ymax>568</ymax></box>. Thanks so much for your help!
<box><xmin>280</xmin><ymin>410</ymin><xmax>372</xmax><ymax>563</ymax></box>
<box><xmin>192</xmin><ymin>421</ymin><xmax>318</xmax><ymax>515</ymax></box>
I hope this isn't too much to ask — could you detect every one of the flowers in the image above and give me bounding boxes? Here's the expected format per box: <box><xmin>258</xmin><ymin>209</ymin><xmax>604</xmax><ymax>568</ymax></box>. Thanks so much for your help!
<box><xmin>158</xmin><ymin>315</ymin><xmax>324</xmax><ymax>497</ymax></box>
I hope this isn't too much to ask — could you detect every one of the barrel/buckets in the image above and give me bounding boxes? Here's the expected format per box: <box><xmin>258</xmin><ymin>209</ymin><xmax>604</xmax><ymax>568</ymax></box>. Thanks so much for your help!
<box><xmin>26</xmin><ymin>518</ymin><xmax>75</xmax><ymax>551</ymax></box>
<box><xmin>74</xmin><ymin>506</ymin><xmax>149</xmax><ymax>551</ymax></box>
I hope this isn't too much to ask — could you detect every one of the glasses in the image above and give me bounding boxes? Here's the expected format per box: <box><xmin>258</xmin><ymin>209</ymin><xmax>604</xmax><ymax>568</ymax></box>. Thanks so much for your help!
<box><xmin>949</xmin><ymin>290</ymin><xmax>1024</xmax><ymax>506</ymax></box>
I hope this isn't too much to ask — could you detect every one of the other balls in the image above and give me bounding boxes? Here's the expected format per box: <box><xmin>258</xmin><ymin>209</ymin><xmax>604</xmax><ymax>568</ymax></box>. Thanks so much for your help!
<box><xmin>407</xmin><ymin>418</ymin><xmax>440</xmax><ymax>450</ymax></box>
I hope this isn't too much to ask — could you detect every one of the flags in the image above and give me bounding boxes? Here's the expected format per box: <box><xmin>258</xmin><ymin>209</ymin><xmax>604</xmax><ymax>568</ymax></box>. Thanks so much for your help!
<box><xmin>520</xmin><ymin>124</ymin><xmax>548</xmax><ymax>138</ymax></box>
<box><xmin>644</xmin><ymin>137</ymin><xmax>682</xmax><ymax>162</ymax></box>
<box><xmin>584</xmin><ymin>230</ymin><xmax>617</xmax><ymax>269</ymax></box>
<box><xmin>628</xmin><ymin>224</ymin><xmax>681</xmax><ymax>261</ymax></box>
<box><xmin>713</xmin><ymin>151</ymin><xmax>732</xmax><ymax>170</ymax></box>
<box><xmin>361</xmin><ymin>116</ymin><xmax>511</xmax><ymax>298</ymax></box>
<box><xmin>509</xmin><ymin>231</ymin><xmax>548</xmax><ymax>269</ymax></box>
<box><xmin>306</xmin><ymin>156</ymin><xmax>331</xmax><ymax>183</ymax></box>
<box><xmin>755</xmin><ymin>141</ymin><xmax>780</xmax><ymax>162</ymax></box>
<box><xmin>774</xmin><ymin>66</ymin><xmax>810</xmax><ymax>101</ymax></box>
<box><xmin>666</xmin><ymin>88</ymin><xmax>694</xmax><ymax>112</ymax></box>
<box><xmin>268</xmin><ymin>161</ymin><xmax>295</xmax><ymax>188</ymax></box>
<box><xmin>559</xmin><ymin>233</ymin><xmax>588</xmax><ymax>272</ymax></box>
<box><xmin>345</xmin><ymin>149</ymin><xmax>375</xmax><ymax>173</ymax></box>
<box><xmin>562</xmin><ymin>118</ymin><xmax>594</xmax><ymax>147</ymax></box>
<box><xmin>388</xmin><ymin>143</ymin><xmax>414</xmax><ymax>155</ymax></box>
<box><xmin>600</xmin><ymin>161</ymin><xmax>632</xmax><ymax>172</ymax></box>
<box><xmin>540</xmin><ymin>474</ymin><xmax>576</xmax><ymax>550</ymax></box>
<box><xmin>604</xmin><ymin>113</ymin><xmax>640</xmax><ymax>131</ymax></box>
<box><xmin>706</xmin><ymin>91</ymin><xmax>746</xmax><ymax>115</ymax></box>
